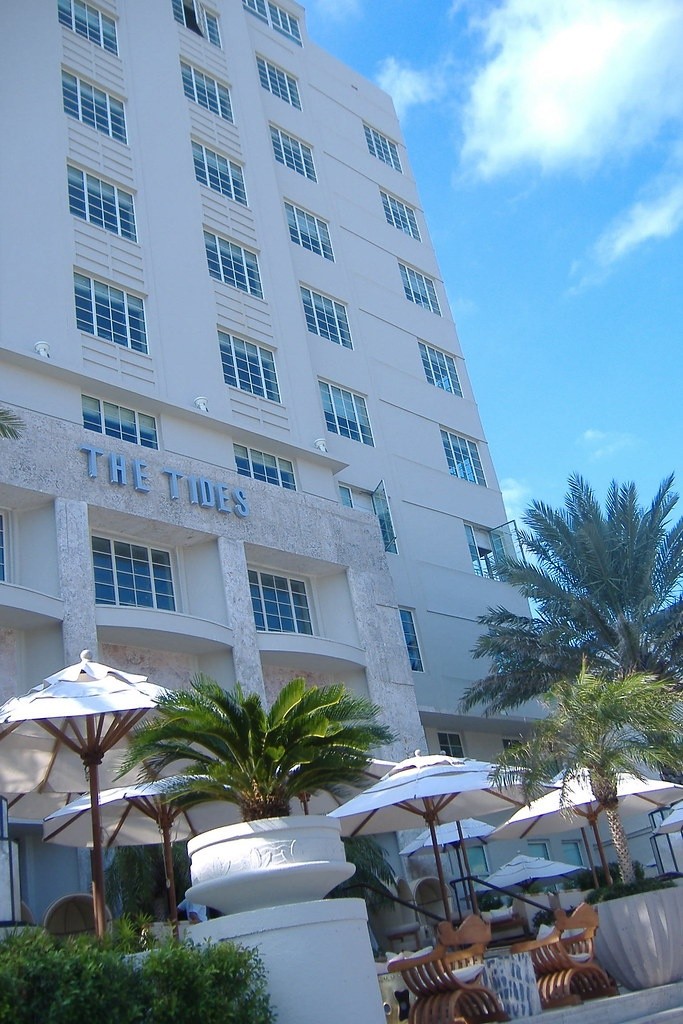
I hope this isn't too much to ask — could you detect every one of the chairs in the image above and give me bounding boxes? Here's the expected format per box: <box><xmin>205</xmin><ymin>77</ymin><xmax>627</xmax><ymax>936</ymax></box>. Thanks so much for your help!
<box><xmin>387</xmin><ymin>902</ymin><xmax>614</xmax><ymax>1024</ymax></box>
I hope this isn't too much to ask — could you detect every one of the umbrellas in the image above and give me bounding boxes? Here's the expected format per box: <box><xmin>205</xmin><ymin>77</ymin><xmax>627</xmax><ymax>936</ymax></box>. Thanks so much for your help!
<box><xmin>0</xmin><ymin>650</ymin><xmax>683</xmax><ymax>939</ymax></box>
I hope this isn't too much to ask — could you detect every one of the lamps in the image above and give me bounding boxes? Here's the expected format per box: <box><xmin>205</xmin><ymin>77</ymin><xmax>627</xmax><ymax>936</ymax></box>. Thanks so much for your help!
<box><xmin>314</xmin><ymin>437</ymin><xmax>328</xmax><ymax>453</ymax></box>
<box><xmin>33</xmin><ymin>341</ymin><xmax>51</xmax><ymax>358</ymax></box>
<box><xmin>193</xmin><ymin>396</ymin><xmax>210</xmax><ymax>414</ymax></box>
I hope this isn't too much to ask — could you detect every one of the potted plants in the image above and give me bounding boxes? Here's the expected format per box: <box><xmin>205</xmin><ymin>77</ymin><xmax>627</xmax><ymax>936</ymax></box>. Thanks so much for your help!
<box><xmin>111</xmin><ymin>672</ymin><xmax>402</xmax><ymax>914</ymax></box>
<box><xmin>583</xmin><ymin>877</ymin><xmax>683</xmax><ymax>990</ymax></box>
